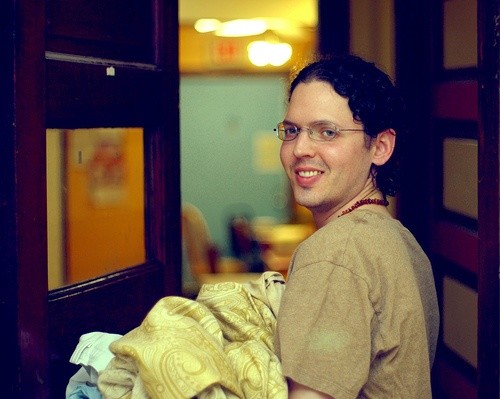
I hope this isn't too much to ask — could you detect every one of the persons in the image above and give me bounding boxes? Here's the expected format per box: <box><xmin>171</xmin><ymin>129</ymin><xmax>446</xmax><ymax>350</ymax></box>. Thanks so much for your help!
<box><xmin>273</xmin><ymin>56</ymin><xmax>441</xmax><ymax>399</ymax></box>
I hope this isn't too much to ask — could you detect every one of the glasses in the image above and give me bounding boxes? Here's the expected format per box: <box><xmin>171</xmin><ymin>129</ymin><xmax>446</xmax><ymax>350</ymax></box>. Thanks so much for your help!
<box><xmin>273</xmin><ymin>121</ymin><xmax>364</xmax><ymax>142</ymax></box>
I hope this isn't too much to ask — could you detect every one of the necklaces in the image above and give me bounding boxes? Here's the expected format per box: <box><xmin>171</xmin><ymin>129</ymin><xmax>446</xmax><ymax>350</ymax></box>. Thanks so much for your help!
<box><xmin>339</xmin><ymin>198</ymin><xmax>390</xmax><ymax>216</ymax></box>
